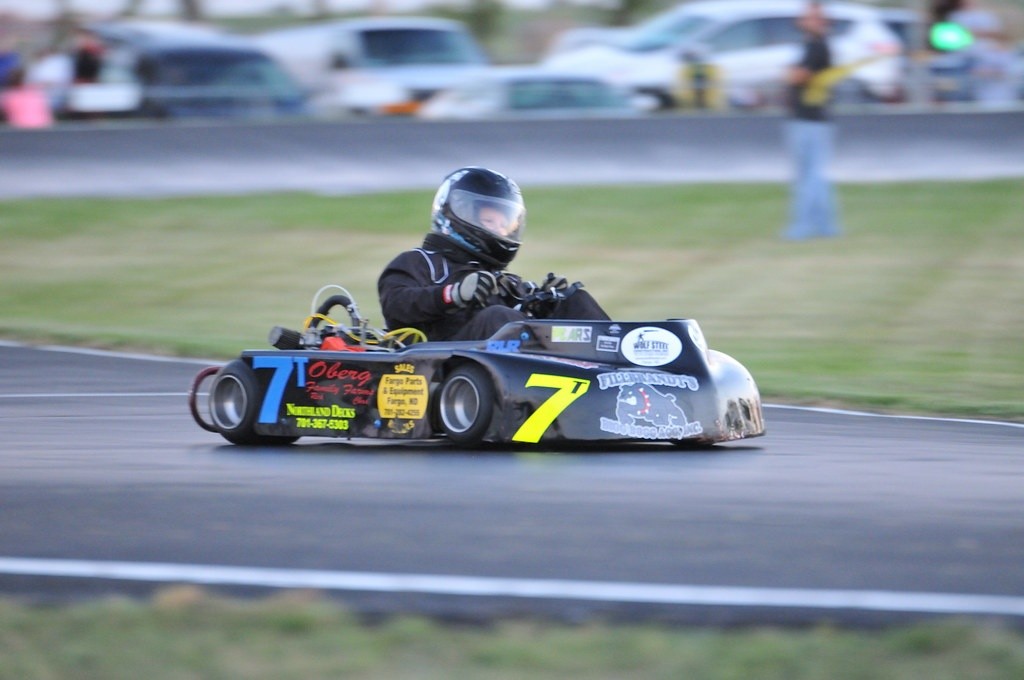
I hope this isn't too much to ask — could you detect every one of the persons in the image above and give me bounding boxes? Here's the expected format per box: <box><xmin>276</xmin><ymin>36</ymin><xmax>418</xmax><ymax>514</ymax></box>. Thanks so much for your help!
<box><xmin>780</xmin><ymin>0</ymin><xmax>836</xmax><ymax>239</ymax></box>
<box><xmin>377</xmin><ymin>166</ymin><xmax>612</xmax><ymax>343</ymax></box>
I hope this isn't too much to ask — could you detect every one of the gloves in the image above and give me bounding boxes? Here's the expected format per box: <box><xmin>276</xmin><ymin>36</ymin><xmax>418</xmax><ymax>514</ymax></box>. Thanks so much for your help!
<box><xmin>541</xmin><ymin>272</ymin><xmax>568</xmax><ymax>295</ymax></box>
<box><xmin>450</xmin><ymin>270</ymin><xmax>500</xmax><ymax>310</ymax></box>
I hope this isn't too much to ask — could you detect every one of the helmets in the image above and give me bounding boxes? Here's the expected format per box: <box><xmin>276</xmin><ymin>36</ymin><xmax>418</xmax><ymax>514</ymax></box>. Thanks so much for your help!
<box><xmin>429</xmin><ymin>167</ymin><xmax>526</xmax><ymax>267</ymax></box>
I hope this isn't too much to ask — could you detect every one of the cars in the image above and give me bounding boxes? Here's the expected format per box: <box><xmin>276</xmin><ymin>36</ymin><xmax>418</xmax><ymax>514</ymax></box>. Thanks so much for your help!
<box><xmin>19</xmin><ymin>28</ymin><xmax>316</xmax><ymax>133</ymax></box>
<box><xmin>243</xmin><ymin>0</ymin><xmax>1024</xmax><ymax>125</ymax></box>
